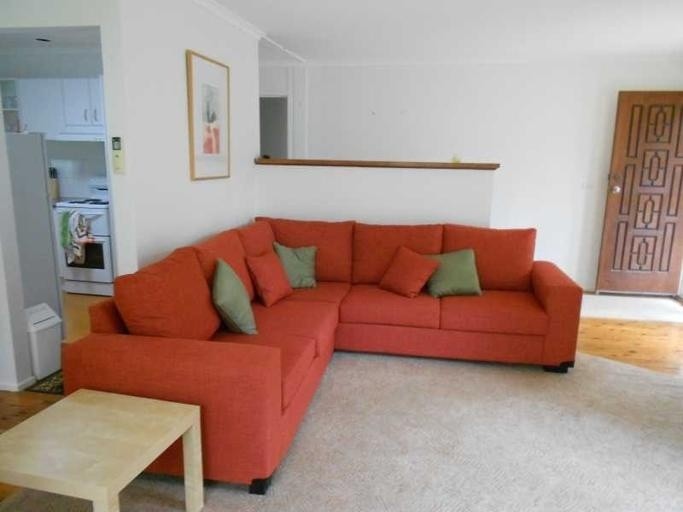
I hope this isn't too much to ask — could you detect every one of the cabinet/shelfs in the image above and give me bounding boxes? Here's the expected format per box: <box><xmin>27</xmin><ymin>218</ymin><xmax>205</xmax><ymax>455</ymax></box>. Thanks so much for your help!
<box><xmin>0</xmin><ymin>76</ymin><xmax>105</xmax><ymax>145</ymax></box>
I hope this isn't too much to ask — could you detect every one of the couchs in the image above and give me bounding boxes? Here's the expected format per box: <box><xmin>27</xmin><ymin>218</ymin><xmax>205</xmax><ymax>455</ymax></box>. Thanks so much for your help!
<box><xmin>59</xmin><ymin>215</ymin><xmax>585</xmax><ymax>496</ymax></box>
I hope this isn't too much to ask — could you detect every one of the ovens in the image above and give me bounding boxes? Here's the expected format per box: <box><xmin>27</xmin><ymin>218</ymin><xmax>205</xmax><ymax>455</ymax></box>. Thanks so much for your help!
<box><xmin>52</xmin><ymin>208</ymin><xmax>115</xmax><ymax>297</ymax></box>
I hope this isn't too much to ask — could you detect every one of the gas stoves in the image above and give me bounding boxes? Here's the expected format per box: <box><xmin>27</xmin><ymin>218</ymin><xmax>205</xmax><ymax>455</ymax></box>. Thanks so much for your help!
<box><xmin>55</xmin><ymin>198</ymin><xmax>109</xmax><ymax>208</ymax></box>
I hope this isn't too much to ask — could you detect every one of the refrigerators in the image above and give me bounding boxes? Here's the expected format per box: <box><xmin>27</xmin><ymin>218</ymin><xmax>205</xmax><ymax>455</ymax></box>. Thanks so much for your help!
<box><xmin>6</xmin><ymin>130</ymin><xmax>68</xmax><ymax>343</ymax></box>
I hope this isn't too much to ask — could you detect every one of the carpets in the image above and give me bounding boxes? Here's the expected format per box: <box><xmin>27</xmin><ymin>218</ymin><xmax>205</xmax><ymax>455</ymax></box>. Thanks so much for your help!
<box><xmin>0</xmin><ymin>348</ymin><xmax>683</xmax><ymax>511</ymax></box>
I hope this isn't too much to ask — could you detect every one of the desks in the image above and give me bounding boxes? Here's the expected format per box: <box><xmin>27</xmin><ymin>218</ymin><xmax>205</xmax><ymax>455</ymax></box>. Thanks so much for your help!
<box><xmin>0</xmin><ymin>387</ymin><xmax>206</xmax><ymax>511</ymax></box>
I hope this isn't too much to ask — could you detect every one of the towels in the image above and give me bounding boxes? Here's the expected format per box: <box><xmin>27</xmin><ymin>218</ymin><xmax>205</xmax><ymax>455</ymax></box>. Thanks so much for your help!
<box><xmin>59</xmin><ymin>208</ymin><xmax>96</xmax><ymax>265</ymax></box>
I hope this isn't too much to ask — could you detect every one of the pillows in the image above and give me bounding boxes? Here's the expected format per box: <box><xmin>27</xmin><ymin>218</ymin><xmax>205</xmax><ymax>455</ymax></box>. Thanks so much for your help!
<box><xmin>209</xmin><ymin>256</ymin><xmax>260</xmax><ymax>335</ymax></box>
<box><xmin>427</xmin><ymin>247</ymin><xmax>486</xmax><ymax>298</ymax></box>
<box><xmin>270</xmin><ymin>239</ymin><xmax>321</xmax><ymax>290</ymax></box>
<box><xmin>245</xmin><ymin>247</ymin><xmax>295</xmax><ymax>308</ymax></box>
<box><xmin>376</xmin><ymin>245</ymin><xmax>442</xmax><ymax>299</ymax></box>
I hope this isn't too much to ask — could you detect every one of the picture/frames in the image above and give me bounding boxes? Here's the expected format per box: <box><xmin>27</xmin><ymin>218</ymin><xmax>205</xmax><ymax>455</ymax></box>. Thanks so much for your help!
<box><xmin>184</xmin><ymin>48</ymin><xmax>231</xmax><ymax>183</ymax></box>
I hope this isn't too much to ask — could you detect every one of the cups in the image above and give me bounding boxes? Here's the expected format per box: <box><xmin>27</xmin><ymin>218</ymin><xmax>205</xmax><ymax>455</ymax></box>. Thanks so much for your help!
<box><xmin>49</xmin><ymin>178</ymin><xmax>59</xmax><ymax>202</ymax></box>
